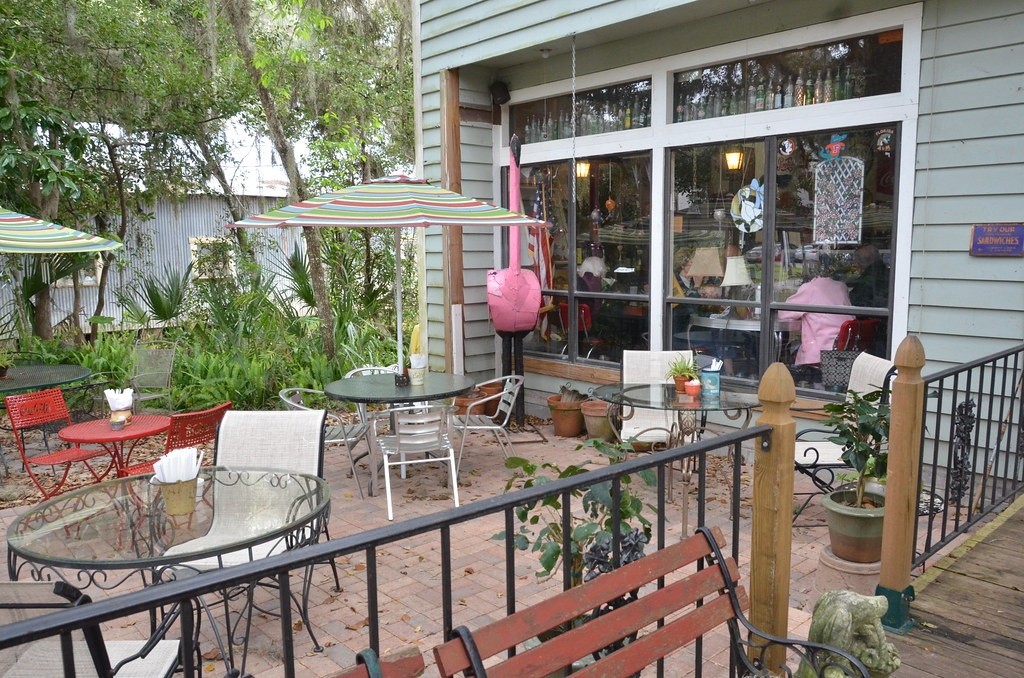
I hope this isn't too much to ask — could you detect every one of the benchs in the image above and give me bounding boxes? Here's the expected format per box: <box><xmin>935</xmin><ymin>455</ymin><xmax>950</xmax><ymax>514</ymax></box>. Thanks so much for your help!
<box><xmin>333</xmin><ymin>526</ymin><xmax>871</xmax><ymax>678</ymax></box>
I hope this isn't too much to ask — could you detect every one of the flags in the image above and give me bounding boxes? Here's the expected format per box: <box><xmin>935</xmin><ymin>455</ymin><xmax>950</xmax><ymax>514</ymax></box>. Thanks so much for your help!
<box><xmin>527</xmin><ymin>172</ymin><xmax>558</xmax><ymax>341</ymax></box>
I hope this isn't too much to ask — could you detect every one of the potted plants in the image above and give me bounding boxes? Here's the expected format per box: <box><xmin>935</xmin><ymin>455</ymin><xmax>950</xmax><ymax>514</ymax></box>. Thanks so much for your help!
<box><xmin>487</xmin><ymin>429</ymin><xmax>674</xmax><ymax>661</ymax></box>
<box><xmin>665</xmin><ymin>356</ymin><xmax>701</xmax><ymax>392</ymax></box>
<box><xmin>822</xmin><ymin>386</ymin><xmax>941</xmax><ymax>559</ymax></box>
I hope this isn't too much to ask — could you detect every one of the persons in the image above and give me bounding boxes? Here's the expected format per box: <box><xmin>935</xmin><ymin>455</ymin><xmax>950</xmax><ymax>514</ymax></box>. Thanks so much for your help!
<box><xmin>778</xmin><ymin>252</ymin><xmax>857</xmax><ymax>366</ymax></box>
<box><xmin>577</xmin><ymin>256</ymin><xmax>617</xmax><ymax>339</ymax></box>
<box><xmin>673</xmin><ymin>243</ymin><xmax>755</xmax><ymax>375</ymax></box>
<box><xmin>849</xmin><ymin>245</ymin><xmax>890</xmax><ymax>319</ymax></box>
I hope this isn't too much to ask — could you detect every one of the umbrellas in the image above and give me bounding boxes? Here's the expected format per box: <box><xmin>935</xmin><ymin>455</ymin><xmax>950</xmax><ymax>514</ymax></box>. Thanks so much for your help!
<box><xmin>0</xmin><ymin>204</ymin><xmax>123</xmax><ymax>254</ymax></box>
<box><xmin>224</xmin><ymin>171</ymin><xmax>553</xmax><ymax>375</ymax></box>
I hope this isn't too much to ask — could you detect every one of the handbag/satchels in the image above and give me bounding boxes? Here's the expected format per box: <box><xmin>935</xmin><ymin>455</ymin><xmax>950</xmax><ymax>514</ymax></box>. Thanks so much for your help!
<box><xmin>820</xmin><ymin>317</ymin><xmax>862</xmax><ymax>388</ymax></box>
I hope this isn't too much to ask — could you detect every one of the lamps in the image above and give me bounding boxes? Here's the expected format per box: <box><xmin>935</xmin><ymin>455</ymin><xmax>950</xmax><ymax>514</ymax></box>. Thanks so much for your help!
<box><xmin>576</xmin><ymin>160</ymin><xmax>590</xmax><ymax>178</ymax></box>
<box><xmin>725</xmin><ymin>142</ymin><xmax>744</xmax><ymax>171</ymax></box>
<box><xmin>684</xmin><ymin>247</ymin><xmax>726</xmax><ymax>311</ymax></box>
<box><xmin>721</xmin><ymin>256</ymin><xmax>752</xmax><ymax>319</ymax></box>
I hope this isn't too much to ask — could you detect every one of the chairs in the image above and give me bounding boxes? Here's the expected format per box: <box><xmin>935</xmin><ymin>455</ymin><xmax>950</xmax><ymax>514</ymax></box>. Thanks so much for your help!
<box><xmin>793</xmin><ymin>319</ymin><xmax>883</xmax><ymax>387</ymax></box>
<box><xmin>0</xmin><ymin>339</ymin><xmax>527</xmax><ymax>678</ymax></box>
<box><xmin>794</xmin><ymin>351</ymin><xmax>906</xmax><ymax>525</ymax></box>
<box><xmin>558</xmin><ymin>302</ymin><xmax>602</xmax><ymax>361</ymax></box>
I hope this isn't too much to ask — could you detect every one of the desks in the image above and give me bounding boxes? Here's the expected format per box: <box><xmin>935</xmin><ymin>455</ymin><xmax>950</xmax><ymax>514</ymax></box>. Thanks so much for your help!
<box><xmin>598</xmin><ymin>304</ymin><xmax>662</xmax><ymax>358</ymax></box>
<box><xmin>592</xmin><ymin>386</ymin><xmax>763</xmax><ymax>503</ymax></box>
<box><xmin>0</xmin><ymin>470</ymin><xmax>332</xmax><ymax>678</ymax></box>
<box><xmin>324</xmin><ymin>372</ymin><xmax>475</xmax><ymax>496</ymax></box>
<box><xmin>0</xmin><ymin>364</ymin><xmax>91</xmax><ymax>475</ymax></box>
<box><xmin>59</xmin><ymin>414</ymin><xmax>171</xmax><ymax>552</ymax></box>
<box><xmin>685</xmin><ymin>312</ymin><xmax>803</xmax><ymax>382</ymax></box>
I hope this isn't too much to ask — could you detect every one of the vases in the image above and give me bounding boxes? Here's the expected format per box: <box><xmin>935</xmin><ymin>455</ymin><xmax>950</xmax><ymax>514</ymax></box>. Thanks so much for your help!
<box><xmin>450</xmin><ymin>388</ymin><xmax>487</xmax><ymax>417</ymax></box>
<box><xmin>581</xmin><ymin>399</ymin><xmax>617</xmax><ymax>440</ymax></box>
<box><xmin>479</xmin><ymin>381</ymin><xmax>507</xmax><ymax>417</ymax></box>
<box><xmin>547</xmin><ymin>395</ymin><xmax>590</xmax><ymax>437</ymax></box>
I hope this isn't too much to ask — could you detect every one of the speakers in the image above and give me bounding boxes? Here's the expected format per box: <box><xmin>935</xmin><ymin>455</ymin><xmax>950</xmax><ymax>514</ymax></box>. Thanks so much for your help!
<box><xmin>490</xmin><ymin>81</ymin><xmax>510</xmax><ymax>105</ymax></box>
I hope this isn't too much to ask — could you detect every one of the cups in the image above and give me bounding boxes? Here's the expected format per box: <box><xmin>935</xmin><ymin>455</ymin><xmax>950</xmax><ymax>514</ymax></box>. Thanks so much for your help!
<box><xmin>407</xmin><ymin>366</ymin><xmax>426</xmax><ymax>385</ymax></box>
<box><xmin>110</xmin><ymin>409</ymin><xmax>133</xmax><ymax>426</ymax></box>
<box><xmin>149</xmin><ymin>476</ymin><xmax>199</xmax><ymax>515</ymax></box>
<box><xmin>111</xmin><ymin>417</ymin><xmax>125</xmax><ymax>431</ymax></box>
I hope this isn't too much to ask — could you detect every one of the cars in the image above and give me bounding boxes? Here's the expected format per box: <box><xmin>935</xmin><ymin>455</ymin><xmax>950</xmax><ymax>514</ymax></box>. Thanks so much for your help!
<box><xmin>746</xmin><ymin>241</ymin><xmax>860</xmax><ymax>263</ymax></box>
